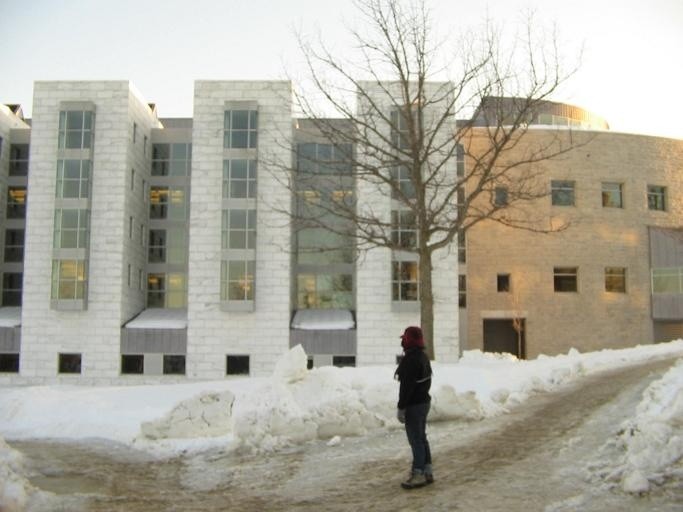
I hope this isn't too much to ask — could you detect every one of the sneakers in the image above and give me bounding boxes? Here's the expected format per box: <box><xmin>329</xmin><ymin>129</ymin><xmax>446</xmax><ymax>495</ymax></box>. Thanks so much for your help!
<box><xmin>401</xmin><ymin>473</ymin><xmax>433</xmax><ymax>489</ymax></box>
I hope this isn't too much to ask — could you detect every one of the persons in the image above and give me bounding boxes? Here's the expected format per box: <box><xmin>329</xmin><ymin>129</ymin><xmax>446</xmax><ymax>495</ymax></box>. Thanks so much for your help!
<box><xmin>393</xmin><ymin>326</ymin><xmax>433</xmax><ymax>489</ymax></box>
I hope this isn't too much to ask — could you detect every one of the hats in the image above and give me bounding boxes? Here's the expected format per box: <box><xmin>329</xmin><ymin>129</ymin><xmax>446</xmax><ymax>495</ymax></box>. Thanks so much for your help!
<box><xmin>401</xmin><ymin>327</ymin><xmax>426</xmax><ymax>351</ymax></box>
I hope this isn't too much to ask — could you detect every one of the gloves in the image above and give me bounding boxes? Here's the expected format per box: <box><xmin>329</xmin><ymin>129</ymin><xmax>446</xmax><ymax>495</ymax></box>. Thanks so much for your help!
<box><xmin>397</xmin><ymin>409</ymin><xmax>406</xmax><ymax>423</ymax></box>
